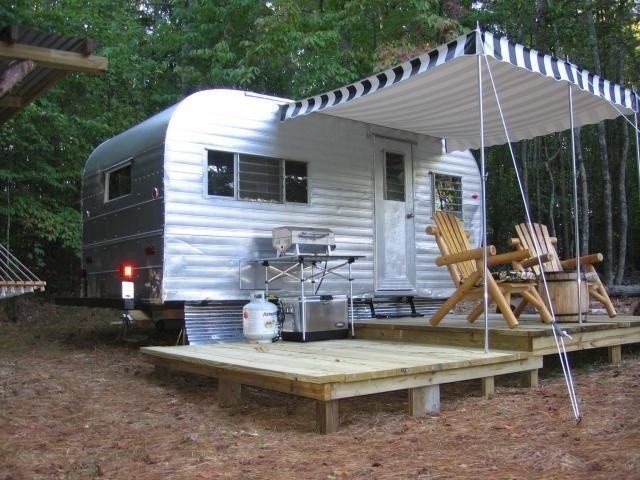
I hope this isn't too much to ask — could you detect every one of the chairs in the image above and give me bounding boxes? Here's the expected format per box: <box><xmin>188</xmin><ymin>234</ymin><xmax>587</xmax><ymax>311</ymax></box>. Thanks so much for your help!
<box><xmin>508</xmin><ymin>223</ymin><xmax>617</xmax><ymax>318</ymax></box>
<box><xmin>425</xmin><ymin>211</ymin><xmax>553</xmax><ymax>329</ymax></box>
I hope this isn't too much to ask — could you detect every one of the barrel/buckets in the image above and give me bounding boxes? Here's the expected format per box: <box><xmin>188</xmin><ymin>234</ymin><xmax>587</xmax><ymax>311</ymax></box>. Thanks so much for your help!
<box><xmin>538</xmin><ymin>270</ymin><xmax>590</xmax><ymax>323</ymax></box>
